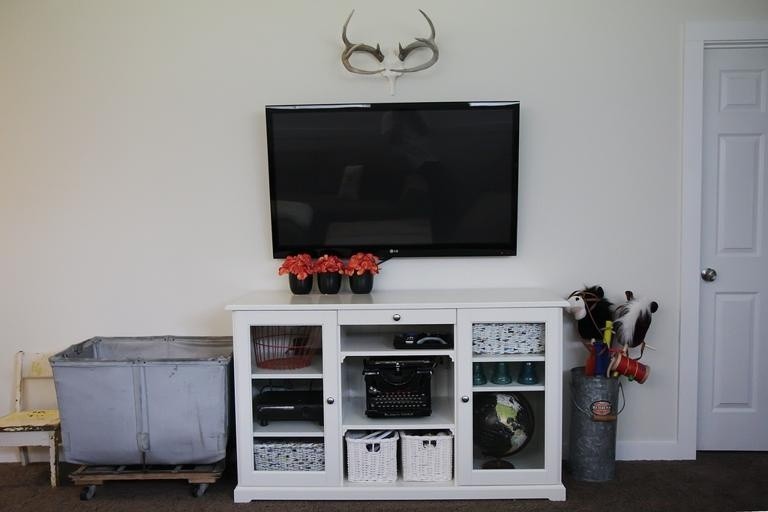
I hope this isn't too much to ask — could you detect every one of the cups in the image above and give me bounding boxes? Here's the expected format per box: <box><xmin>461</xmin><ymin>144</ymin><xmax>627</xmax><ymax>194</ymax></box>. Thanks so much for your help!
<box><xmin>473</xmin><ymin>362</ymin><xmax>538</xmax><ymax>386</ymax></box>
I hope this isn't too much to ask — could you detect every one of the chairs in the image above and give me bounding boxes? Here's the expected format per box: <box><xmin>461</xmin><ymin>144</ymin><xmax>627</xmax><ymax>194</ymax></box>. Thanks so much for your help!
<box><xmin>0</xmin><ymin>351</ymin><xmax>62</xmax><ymax>489</ymax></box>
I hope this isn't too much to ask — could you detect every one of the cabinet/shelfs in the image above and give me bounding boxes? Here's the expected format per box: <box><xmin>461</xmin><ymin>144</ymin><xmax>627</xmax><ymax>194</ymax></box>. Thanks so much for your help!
<box><xmin>224</xmin><ymin>290</ymin><xmax>571</xmax><ymax>504</ymax></box>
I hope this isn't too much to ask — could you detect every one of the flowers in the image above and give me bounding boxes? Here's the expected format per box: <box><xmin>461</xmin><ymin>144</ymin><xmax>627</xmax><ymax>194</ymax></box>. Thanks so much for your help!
<box><xmin>277</xmin><ymin>252</ymin><xmax>382</xmax><ymax>281</ymax></box>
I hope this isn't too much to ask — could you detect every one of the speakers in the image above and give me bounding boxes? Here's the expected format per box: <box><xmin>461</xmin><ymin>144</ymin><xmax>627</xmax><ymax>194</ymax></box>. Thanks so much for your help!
<box><xmin>360</xmin><ymin>358</ymin><xmax>433</xmax><ymax>418</ymax></box>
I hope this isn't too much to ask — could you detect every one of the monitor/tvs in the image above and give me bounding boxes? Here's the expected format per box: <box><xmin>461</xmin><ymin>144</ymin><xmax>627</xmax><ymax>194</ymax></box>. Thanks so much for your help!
<box><xmin>265</xmin><ymin>101</ymin><xmax>520</xmax><ymax>259</ymax></box>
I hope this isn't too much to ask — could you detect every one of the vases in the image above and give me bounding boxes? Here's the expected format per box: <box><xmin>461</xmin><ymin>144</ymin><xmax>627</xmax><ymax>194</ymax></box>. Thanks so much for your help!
<box><xmin>289</xmin><ymin>271</ymin><xmax>373</xmax><ymax>294</ymax></box>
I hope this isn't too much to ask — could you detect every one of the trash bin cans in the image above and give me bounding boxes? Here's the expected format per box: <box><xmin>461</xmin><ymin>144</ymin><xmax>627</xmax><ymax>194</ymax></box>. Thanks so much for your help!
<box><xmin>48</xmin><ymin>336</ymin><xmax>234</xmax><ymax>466</ymax></box>
